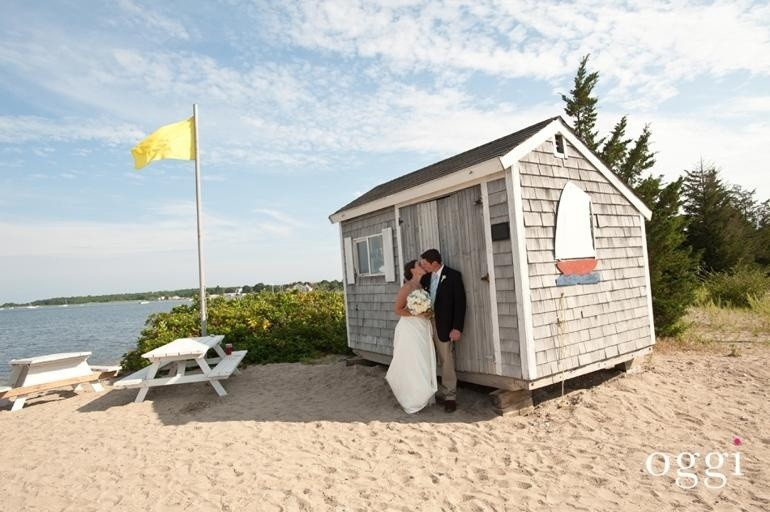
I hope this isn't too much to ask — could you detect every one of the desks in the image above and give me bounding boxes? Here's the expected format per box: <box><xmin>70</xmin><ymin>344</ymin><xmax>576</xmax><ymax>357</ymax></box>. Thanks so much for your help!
<box><xmin>9</xmin><ymin>351</ymin><xmax>104</xmax><ymax>411</ymax></box>
<box><xmin>134</xmin><ymin>336</ymin><xmax>242</xmax><ymax>400</ymax></box>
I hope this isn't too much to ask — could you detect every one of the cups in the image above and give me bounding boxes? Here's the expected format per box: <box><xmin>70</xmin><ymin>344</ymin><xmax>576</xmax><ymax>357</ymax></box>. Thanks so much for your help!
<box><xmin>225</xmin><ymin>343</ymin><xmax>232</xmax><ymax>355</ymax></box>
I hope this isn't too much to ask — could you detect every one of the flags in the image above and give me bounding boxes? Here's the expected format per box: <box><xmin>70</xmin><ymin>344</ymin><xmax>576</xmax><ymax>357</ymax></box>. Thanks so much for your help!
<box><xmin>128</xmin><ymin>113</ymin><xmax>196</xmax><ymax>170</ymax></box>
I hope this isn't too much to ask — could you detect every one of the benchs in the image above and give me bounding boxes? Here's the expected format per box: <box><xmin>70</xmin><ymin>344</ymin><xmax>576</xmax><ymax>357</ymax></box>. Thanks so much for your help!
<box><xmin>0</xmin><ymin>365</ymin><xmax>122</xmax><ymax>411</ymax></box>
<box><xmin>113</xmin><ymin>349</ymin><xmax>171</xmax><ymax>388</ymax></box>
<box><xmin>208</xmin><ymin>349</ymin><xmax>248</xmax><ymax>378</ymax></box>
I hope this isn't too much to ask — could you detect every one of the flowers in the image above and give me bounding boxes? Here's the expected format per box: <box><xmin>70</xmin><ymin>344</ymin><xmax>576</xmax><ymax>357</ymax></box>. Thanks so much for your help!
<box><xmin>441</xmin><ymin>275</ymin><xmax>446</xmax><ymax>282</ymax></box>
<box><xmin>406</xmin><ymin>288</ymin><xmax>436</xmax><ymax>320</ymax></box>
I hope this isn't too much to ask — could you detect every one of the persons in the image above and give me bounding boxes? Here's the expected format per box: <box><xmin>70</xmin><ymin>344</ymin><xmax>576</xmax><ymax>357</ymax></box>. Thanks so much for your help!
<box><xmin>384</xmin><ymin>259</ymin><xmax>439</xmax><ymax>416</ymax></box>
<box><xmin>403</xmin><ymin>249</ymin><xmax>466</xmax><ymax>413</ymax></box>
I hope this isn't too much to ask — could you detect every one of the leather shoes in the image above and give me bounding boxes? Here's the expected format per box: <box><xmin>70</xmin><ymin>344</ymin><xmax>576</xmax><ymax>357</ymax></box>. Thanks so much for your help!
<box><xmin>445</xmin><ymin>401</ymin><xmax>456</xmax><ymax>412</ymax></box>
